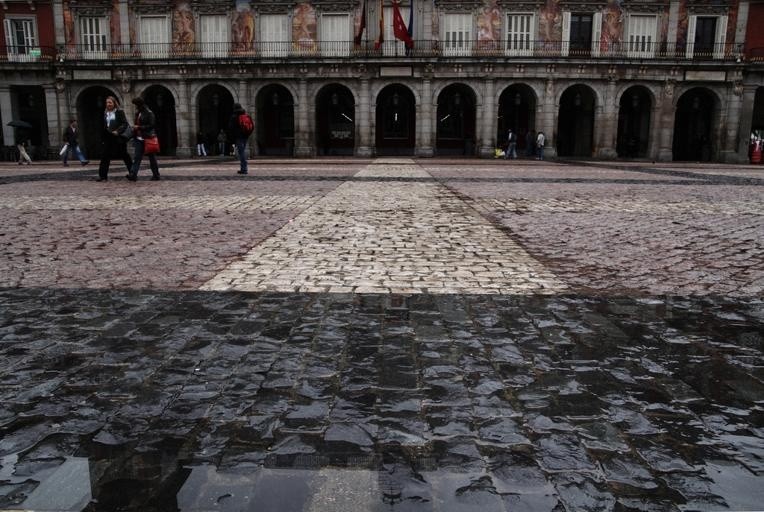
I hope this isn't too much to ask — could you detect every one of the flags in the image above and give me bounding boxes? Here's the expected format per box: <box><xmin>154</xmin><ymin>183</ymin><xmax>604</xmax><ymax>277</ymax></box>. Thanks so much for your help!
<box><xmin>351</xmin><ymin>1</ymin><xmax>414</xmax><ymax>58</ymax></box>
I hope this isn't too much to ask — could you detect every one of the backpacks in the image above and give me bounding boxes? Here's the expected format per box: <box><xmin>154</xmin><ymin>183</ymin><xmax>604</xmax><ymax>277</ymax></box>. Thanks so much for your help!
<box><xmin>237</xmin><ymin>112</ymin><xmax>253</xmax><ymax>136</ymax></box>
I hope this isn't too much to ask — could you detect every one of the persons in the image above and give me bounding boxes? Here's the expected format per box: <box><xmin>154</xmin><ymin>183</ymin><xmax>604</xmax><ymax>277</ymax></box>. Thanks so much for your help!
<box><xmin>126</xmin><ymin>97</ymin><xmax>162</xmax><ymax>182</ymax></box>
<box><xmin>7</xmin><ymin>119</ymin><xmax>33</xmax><ymax>164</ymax></box>
<box><xmin>227</xmin><ymin>103</ymin><xmax>253</xmax><ymax>176</ymax></box>
<box><xmin>96</xmin><ymin>96</ymin><xmax>135</xmax><ymax>183</ymax></box>
<box><xmin>195</xmin><ymin>128</ymin><xmax>239</xmax><ymax>161</ymax></box>
<box><xmin>60</xmin><ymin>118</ymin><xmax>89</xmax><ymax>168</ymax></box>
<box><xmin>494</xmin><ymin>127</ymin><xmax>545</xmax><ymax>161</ymax></box>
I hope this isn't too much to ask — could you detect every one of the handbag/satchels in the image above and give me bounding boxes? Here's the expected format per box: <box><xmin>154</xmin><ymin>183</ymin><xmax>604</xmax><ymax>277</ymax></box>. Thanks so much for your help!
<box><xmin>143</xmin><ymin>136</ymin><xmax>160</xmax><ymax>155</ymax></box>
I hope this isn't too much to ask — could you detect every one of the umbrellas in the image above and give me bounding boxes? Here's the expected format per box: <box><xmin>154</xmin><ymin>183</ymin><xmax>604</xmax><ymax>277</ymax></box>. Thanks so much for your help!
<box><xmin>5</xmin><ymin>119</ymin><xmax>31</xmax><ymax>130</ymax></box>
